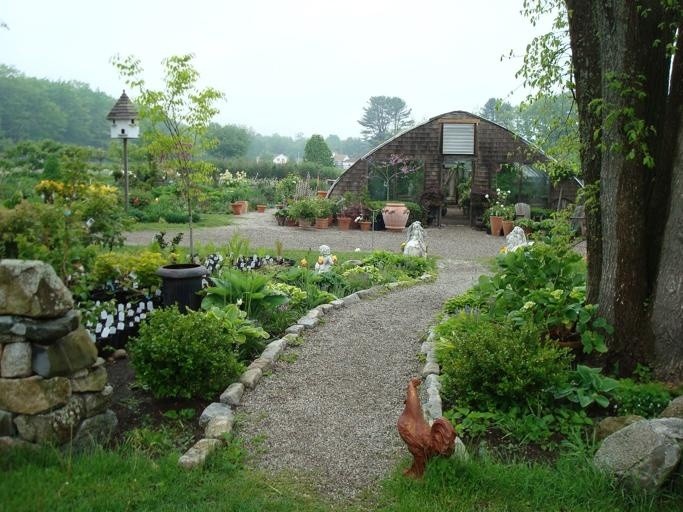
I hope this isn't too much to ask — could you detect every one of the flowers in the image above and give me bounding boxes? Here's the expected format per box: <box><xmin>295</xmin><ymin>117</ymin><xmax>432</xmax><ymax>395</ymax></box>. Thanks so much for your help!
<box><xmin>482</xmin><ymin>186</ymin><xmax>515</xmax><ymax>219</ymax></box>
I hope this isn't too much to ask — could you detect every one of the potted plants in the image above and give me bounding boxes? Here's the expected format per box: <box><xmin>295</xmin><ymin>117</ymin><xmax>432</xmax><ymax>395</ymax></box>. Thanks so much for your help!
<box><xmin>512</xmin><ymin>217</ymin><xmax>532</xmax><ymax>238</ymax></box>
<box><xmin>278</xmin><ymin>190</ymin><xmax>371</xmax><ymax>233</ymax></box>
<box><xmin>275</xmin><ymin>202</ymin><xmax>283</xmax><ymax>210</ymax></box>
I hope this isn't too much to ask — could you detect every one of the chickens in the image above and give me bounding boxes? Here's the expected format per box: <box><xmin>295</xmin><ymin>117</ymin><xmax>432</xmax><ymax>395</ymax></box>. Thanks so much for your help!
<box><xmin>394</xmin><ymin>378</ymin><xmax>457</xmax><ymax>478</ymax></box>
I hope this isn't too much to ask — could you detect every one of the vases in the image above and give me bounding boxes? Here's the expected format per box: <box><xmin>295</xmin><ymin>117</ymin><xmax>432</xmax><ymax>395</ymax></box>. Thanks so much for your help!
<box><xmin>490</xmin><ymin>216</ymin><xmax>502</xmax><ymax>235</ymax></box>
<box><xmin>485</xmin><ymin>222</ymin><xmax>491</xmax><ymax>234</ymax></box>
<box><xmin>235</xmin><ymin>201</ymin><xmax>247</xmax><ymax>213</ymax></box>
<box><xmin>501</xmin><ymin>220</ymin><xmax>513</xmax><ymax>237</ymax></box>
<box><xmin>231</xmin><ymin>203</ymin><xmax>242</xmax><ymax>215</ymax></box>
<box><xmin>256</xmin><ymin>204</ymin><xmax>266</xmax><ymax>212</ymax></box>
